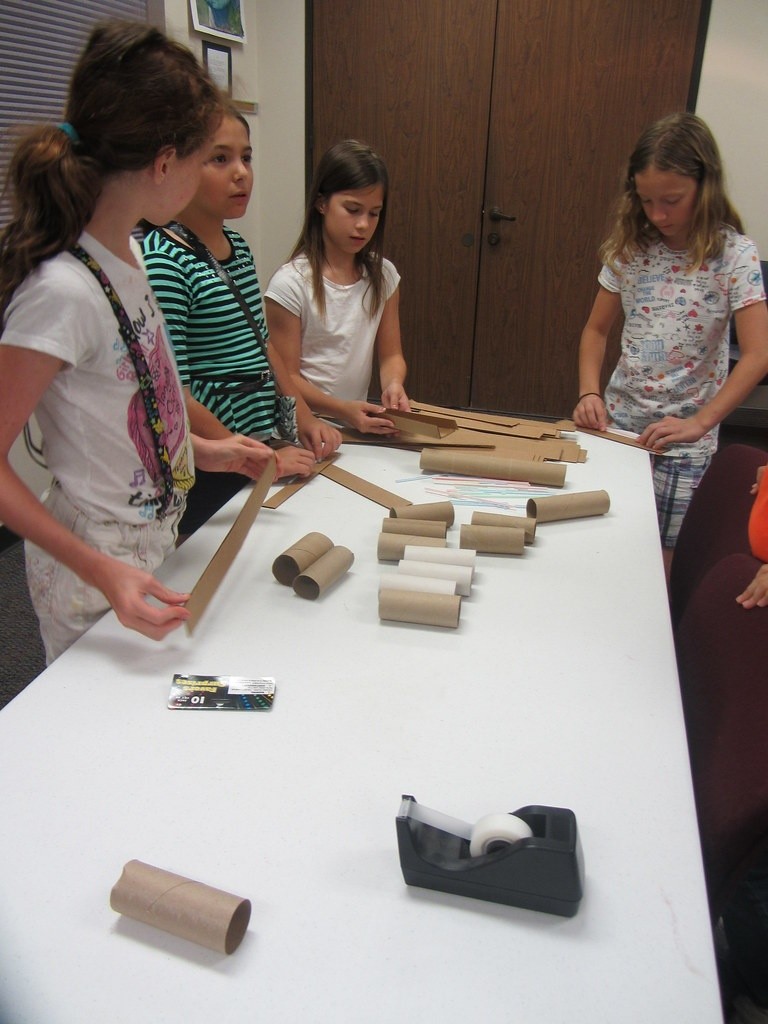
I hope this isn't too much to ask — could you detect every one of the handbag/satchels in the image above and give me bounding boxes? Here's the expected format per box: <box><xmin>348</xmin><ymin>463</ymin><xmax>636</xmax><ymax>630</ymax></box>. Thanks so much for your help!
<box><xmin>273</xmin><ymin>394</ymin><xmax>302</xmax><ymax>448</ymax></box>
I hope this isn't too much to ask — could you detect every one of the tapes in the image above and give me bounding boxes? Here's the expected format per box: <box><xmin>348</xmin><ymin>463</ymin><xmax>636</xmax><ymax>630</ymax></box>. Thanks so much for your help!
<box><xmin>470</xmin><ymin>813</ymin><xmax>533</xmax><ymax>857</ymax></box>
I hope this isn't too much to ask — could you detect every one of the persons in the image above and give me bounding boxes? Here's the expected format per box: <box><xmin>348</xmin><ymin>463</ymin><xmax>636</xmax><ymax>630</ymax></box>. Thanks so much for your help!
<box><xmin>572</xmin><ymin>114</ymin><xmax>768</xmax><ymax>581</ymax></box>
<box><xmin>0</xmin><ymin>18</ymin><xmax>279</xmax><ymax>661</ymax></box>
<box><xmin>138</xmin><ymin>105</ymin><xmax>342</xmax><ymax>538</ymax></box>
<box><xmin>264</xmin><ymin>140</ymin><xmax>412</xmax><ymax>439</ymax></box>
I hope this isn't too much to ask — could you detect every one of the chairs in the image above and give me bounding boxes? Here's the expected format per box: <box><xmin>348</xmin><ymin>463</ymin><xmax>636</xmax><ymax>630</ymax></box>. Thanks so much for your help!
<box><xmin>661</xmin><ymin>552</ymin><xmax>768</xmax><ymax>929</ymax></box>
<box><xmin>665</xmin><ymin>439</ymin><xmax>768</xmax><ymax>622</ymax></box>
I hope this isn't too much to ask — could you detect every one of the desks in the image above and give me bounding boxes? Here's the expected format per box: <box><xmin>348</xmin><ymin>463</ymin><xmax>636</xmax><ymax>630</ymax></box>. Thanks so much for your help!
<box><xmin>0</xmin><ymin>396</ymin><xmax>728</xmax><ymax>1024</ymax></box>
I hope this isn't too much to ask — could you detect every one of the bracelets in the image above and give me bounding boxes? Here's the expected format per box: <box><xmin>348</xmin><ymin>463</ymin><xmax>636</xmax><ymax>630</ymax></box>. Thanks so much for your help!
<box><xmin>577</xmin><ymin>393</ymin><xmax>602</xmax><ymax>402</ymax></box>
